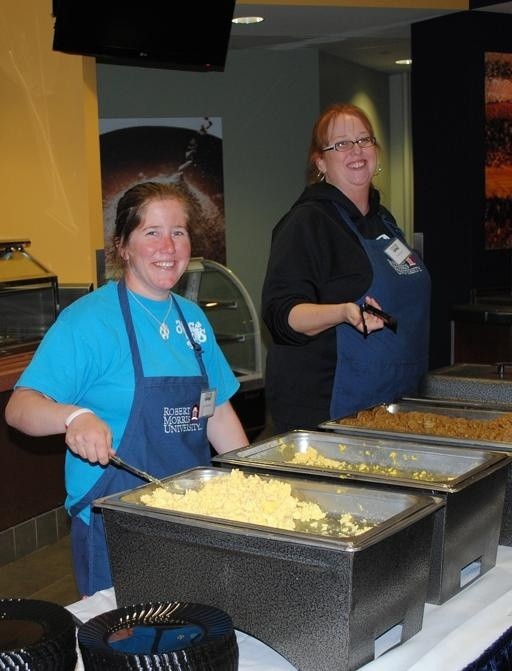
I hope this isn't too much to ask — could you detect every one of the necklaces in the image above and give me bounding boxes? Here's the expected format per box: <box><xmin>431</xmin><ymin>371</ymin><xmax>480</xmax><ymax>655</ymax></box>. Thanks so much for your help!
<box><xmin>125</xmin><ymin>283</ymin><xmax>174</xmax><ymax>341</ymax></box>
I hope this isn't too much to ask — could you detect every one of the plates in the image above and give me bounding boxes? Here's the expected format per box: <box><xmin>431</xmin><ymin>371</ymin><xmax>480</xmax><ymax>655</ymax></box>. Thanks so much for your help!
<box><xmin>78</xmin><ymin>601</ymin><xmax>239</xmax><ymax>671</ymax></box>
<box><xmin>0</xmin><ymin>597</ymin><xmax>78</xmax><ymax>671</ymax></box>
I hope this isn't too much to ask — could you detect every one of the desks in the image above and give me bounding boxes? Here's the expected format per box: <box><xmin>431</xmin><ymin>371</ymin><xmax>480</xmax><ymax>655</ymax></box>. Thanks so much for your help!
<box><xmin>65</xmin><ymin>545</ymin><xmax>512</xmax><ymax>671</ymax></box>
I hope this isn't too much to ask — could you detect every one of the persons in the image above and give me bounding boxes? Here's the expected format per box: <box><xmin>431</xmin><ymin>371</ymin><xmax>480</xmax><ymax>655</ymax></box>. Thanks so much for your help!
<box><xmin>259</xmin><ymin>101</ymin><xmax>435</xmax><ymax>438</ymax></box>
<box><xmin>3</xmin><ymin>179</ymin><xmax>255</xmax><ymax>605</ymax></box>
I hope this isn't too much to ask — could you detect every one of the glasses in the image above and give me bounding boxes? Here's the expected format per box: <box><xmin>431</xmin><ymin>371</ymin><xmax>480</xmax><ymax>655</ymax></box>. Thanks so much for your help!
<box><xmin>320</xmin><ymin>135</ymin><xmax>376</xmax><ymax>152</ymax></box>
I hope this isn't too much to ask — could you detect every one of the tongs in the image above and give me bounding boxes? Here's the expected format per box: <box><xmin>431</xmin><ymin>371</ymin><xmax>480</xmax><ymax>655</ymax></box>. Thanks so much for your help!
<box><xmin>360</xmin><ymin>302</ymin><xmax>398</xmax><ymax>339</ymax></box>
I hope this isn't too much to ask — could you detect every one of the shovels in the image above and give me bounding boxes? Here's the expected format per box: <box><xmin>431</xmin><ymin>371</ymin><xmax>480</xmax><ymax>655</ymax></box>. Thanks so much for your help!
<box><xmin>110</xmin><ymin>455</ymin><xmax>206</xmax><ymax>494</ymax></box>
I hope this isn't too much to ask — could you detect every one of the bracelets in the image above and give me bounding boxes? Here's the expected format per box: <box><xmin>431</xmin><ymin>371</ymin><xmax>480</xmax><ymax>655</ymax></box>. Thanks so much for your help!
<box><xmin>64</xmin><ymin>407</ymin><xmax>97</xmax><ymax>430</ymax></box>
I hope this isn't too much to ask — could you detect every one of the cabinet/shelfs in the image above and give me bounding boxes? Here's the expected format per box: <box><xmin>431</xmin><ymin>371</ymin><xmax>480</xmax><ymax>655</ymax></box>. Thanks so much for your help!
<box><xmin>171</xmin><ymin>256</ymin><xmax>267</xmax><ymax>458</ymax></box>
